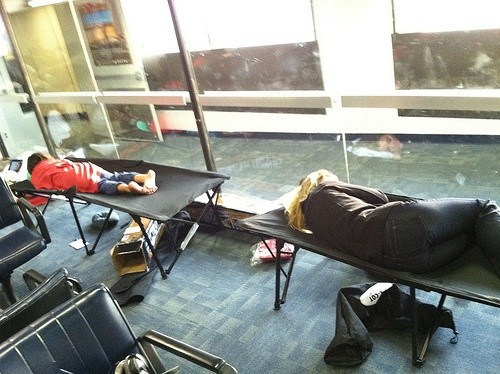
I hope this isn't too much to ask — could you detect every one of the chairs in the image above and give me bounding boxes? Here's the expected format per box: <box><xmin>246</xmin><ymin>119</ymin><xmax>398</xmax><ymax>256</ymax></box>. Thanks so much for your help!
<box><xmin>0</xmin><ymin>172</ymin><xmax>239</xmax><ymax>374</ymax></box>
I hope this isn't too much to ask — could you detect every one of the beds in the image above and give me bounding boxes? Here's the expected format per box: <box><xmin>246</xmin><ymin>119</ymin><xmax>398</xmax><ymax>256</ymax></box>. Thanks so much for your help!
<box><xmin>10</xmin><ymin>158</ymin><xmax>230</xmax><ymax>280</ymax></box>
<box><xmin>233</xmin><ymin>205</ymin><xmax>500</xmax><ymax>368</ymax></box>
